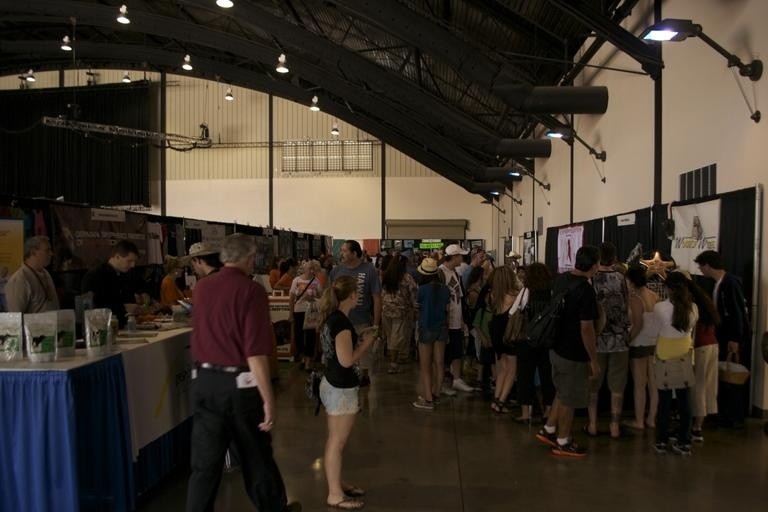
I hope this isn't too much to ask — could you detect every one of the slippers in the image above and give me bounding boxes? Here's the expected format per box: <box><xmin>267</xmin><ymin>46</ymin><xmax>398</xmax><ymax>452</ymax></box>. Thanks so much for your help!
<box><xmin>327</xmin><ymin>481</ymin><xmax>367</xmax><ymax>511</ymax></box>
<box><xmin>584</xmin><ymin>417</ymin><xmax>657</xmax><ymax>437</ymax></box>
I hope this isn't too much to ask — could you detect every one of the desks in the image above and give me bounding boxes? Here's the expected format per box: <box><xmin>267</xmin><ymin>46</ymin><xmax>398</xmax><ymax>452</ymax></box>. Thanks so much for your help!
<box><xmin>1</xmin><ymin>327</ymin><xmax>194</xmax><ymax>512</ymax></box>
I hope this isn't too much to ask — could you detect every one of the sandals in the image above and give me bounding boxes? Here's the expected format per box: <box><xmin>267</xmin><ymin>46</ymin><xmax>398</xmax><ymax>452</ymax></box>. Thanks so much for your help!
<box><xmin>489</xmin><ymin>398</ymin><xmax>550</xmax><ymax>424</ymax></box>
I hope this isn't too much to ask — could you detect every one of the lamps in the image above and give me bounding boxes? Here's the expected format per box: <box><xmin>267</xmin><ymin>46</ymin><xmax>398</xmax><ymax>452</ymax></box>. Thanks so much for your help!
<box><xmin>309</xmin><ymin>95</ymin><xmax>321</xmax><ymax>112</ymax></box>
<box><xmin>480</xmin><ymin>126</ymin><xmax>607</xmax><ymax>224</ymax></box>
<box><xmin>26</xmin><ymin>67</ymin><xmax>35</xmax><ymax>85</ymax></box>
<box><xmin>223</xmin><ymin>85</ymin><xmax>235</xmax><ymax>102</ymax></box>
<box><xmin>59</xmin><ymin>35</ymin><xmax>75</xmax><ymax>53</ymax></box>
<box><xmin>180</xmin><ymin>54</ymin><xmax>194</xmax><ymax>73</ymax></box>
<box><xmin>638</xmin><ymin>18</ymin><xmax>764</xmax><ymax>124</ymax></box>
<box><xmin>114</xmin><ymin>2</ymin><xmax>133</xmax><ymax>26</ymax></box>
<box><xmin>215</xmin><ymin>1</ymin><xmax>236</xmax><ymax>11</ymax></box>
<box><xmin>329</xmin><ymin>126</ymin><xmax>339</xmax><ymax>137</ymax></box>
<box><xmin>275</xmin><ymin>53</ymin><xmax>290</xmax><ymax>74</ymax></box>
<box><xmin>120</xmin><ymin>70</ymin><xmax>133</xmax><ymax>85</ymax></box>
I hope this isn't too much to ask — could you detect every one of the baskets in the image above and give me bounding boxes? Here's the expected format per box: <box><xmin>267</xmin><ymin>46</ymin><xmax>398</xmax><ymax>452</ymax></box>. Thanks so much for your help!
<box><xmin>718</xmin><ymin>350</ymin><xmax>749</xmax><ymax>385</ymax></box>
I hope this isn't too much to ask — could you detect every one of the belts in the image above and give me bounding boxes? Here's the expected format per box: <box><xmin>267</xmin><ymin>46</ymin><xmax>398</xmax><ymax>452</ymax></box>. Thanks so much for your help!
<box><xmin>199</xmin><ymin>362</ymin><xmax>243</xmax><ymax>374</ymax></box>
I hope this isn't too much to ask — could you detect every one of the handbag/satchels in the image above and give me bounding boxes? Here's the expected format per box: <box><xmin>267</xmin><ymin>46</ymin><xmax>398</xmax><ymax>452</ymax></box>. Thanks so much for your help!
<box><xmin>302</xmin><ymin>301</ymin><xmax>322</xmax><ymax>329</ymax></box>
<box><xmin>655</xmin><ymin>352</ymin><xmax>694</xmax><ymax>390</ymax></box>
<box><xmin>303</xmin><ymin>367</ymin><xmax>321</xmax><ymax>401</ymax></box>
<box><xmin>504</xmin><ymin>309</ymin><xmax>528</xmax><ymax>343</ymax></box>
<box><xmin>525</xmin><ymin>296</ymin><xmax>565</xmax><ymax>344</ymax></box>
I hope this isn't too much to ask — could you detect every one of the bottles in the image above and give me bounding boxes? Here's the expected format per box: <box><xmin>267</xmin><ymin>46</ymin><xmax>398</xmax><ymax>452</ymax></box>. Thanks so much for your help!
<box><xmin>128</xmin><ymin>316</ymin><xmax>136</xmax><ymax>333</ymax></box>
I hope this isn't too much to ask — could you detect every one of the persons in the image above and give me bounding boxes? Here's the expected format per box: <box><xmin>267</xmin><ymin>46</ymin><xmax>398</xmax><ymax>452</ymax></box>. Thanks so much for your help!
<box><xmin>159</xmin><ymin>234</ymin><xmax>768</xmax><ymax>511</ymax></box>
<box><xmin>83</xmin><ymin>240</ymin><xmax>140</xmax><ymax>331</ymax></box>
<box><xmin>4</xmin><ymin>236</ymin><xmax>60</xmax><ymax>350</ymax></box>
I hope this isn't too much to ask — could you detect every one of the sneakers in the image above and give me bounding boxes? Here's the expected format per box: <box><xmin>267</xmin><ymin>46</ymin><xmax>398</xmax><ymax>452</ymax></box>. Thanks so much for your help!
<box><xmin>537</xmin><ymin>427</ymin><xmax>588</xmax><ymax>457</ymax></box>
<box><xmin>286</xmin><ymin>502</ymin><xmax>302</xmax><ymax>511</ymax></box>
<box><xmin>389</xmin><ymin>365</ymin><xmax>404</xmax><ymax>374</ymax></box>
<box><xmin>440</xmin><ymin>376</ymin><xmax>496</xmax><ymax>396</ymax></box>
<box><xmin>649</xmin><ymin>426</ymin><xmax>703</xmax><ymax>457</ymax></box>
<box><xmin>410</xmin><ymin>394</ymin><xmax>441</xmax><ymax>410</ymax></box>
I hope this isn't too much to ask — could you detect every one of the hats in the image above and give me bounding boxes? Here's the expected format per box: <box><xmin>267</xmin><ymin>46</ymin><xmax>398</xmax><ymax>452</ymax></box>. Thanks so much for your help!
<box><xmin>506</xmin><ymin>250</ymin><xmax>522</xmax><ymax>259</ymax></box>
<box><xmin>444</xmin><ymin>243</ymin><xmax>470</xmax><ymax>256</ymax></box>
<box><xmin>417</xmin><ymin>258</ymin><xmax>440</xmax><ymax>276</ymax></box>
<box><xmin>183</xmin><ymin>241</ymin><xmax>221</xmax><ymax>263</ymax></box>
